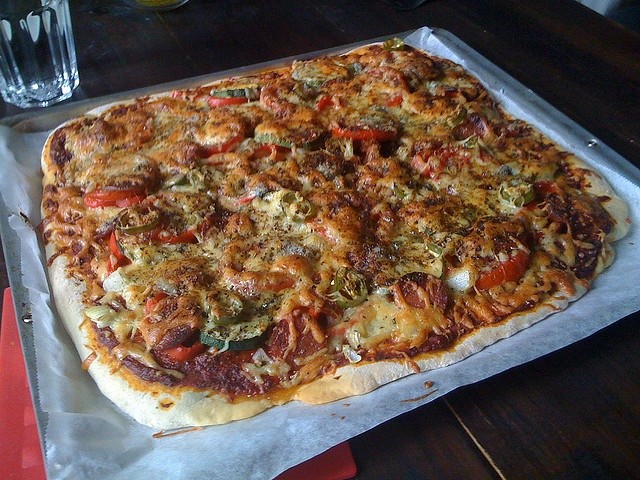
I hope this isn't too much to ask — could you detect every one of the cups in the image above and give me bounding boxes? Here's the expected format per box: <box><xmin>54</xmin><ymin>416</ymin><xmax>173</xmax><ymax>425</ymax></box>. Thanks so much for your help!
<box><xmin>0</xmin><ymin>0</ymin><xmax>80</xmax><ymax>109</ymax></box>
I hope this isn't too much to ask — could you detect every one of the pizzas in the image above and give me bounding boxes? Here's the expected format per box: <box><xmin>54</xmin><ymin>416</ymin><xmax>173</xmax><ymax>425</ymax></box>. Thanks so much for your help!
<box><xmin>39</xmin><ymin>36</ymin><xmax>632</xmax><ymax>433</ymax></box>
